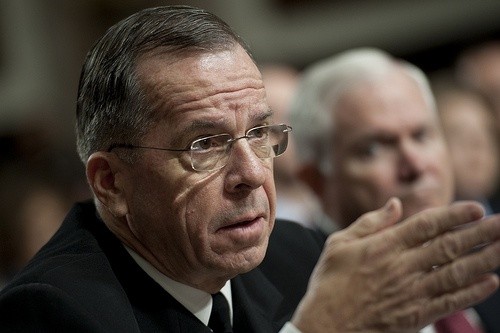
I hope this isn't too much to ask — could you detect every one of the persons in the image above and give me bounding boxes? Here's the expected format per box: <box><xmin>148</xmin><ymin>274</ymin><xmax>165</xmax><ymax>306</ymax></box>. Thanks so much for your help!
<box><xmin>0</xmin><ymin>6</ymin><xmax>500</xmax><ymax>333</ymax></box>
<box><xmin>289</xmin><ymin>48</ymin><xmax>500</xmax><ymax>333</ymax></box>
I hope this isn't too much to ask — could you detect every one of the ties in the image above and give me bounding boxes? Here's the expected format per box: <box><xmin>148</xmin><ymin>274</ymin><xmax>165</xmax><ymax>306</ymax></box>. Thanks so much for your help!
<box><xmin>434</xmin><ymin>308</ymin><xmax>477</xmax><ymax>333</ymax></box>
<box><xmin>208</xmin><ymin>291</ymin><xmax>231</xmax><ymax>333</ymax></box>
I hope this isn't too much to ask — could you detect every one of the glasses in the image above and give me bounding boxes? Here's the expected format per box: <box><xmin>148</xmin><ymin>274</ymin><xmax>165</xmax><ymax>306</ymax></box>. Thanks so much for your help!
<box><xmin>106</xmin><ymin>122</ymin><xmax>294</xmax><ymax>172</ymax></box>
